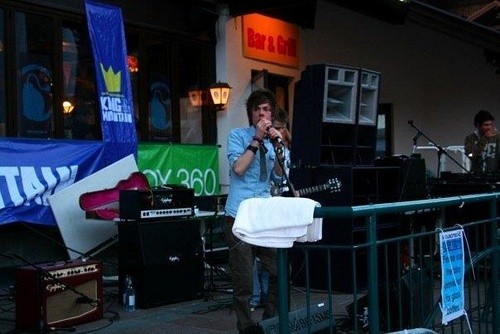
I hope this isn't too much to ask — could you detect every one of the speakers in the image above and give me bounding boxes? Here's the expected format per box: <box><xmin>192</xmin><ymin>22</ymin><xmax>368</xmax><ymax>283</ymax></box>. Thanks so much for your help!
<box><xmin>15</xmin><ymin>260</ymin><xmax>105</xmax><ymax>329</ymax></box>
<box><xmin>116</xmin><ymin>217</ymin><xmax>206</xmax><ymax>310</ymax></box>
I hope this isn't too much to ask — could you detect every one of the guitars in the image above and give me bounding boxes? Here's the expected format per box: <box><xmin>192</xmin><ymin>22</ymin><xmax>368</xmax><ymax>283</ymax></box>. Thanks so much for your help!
<box><xmin>281</xmin><ymin>177</ymin><xmax>342</xmax><ymax>198</ymax></box>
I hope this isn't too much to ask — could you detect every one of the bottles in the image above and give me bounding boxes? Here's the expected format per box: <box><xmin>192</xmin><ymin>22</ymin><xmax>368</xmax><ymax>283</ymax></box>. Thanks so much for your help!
<box><xmin>122</xmin><ymin>275</ymin><xmax>135</xmax><ymax>312</ymax></box>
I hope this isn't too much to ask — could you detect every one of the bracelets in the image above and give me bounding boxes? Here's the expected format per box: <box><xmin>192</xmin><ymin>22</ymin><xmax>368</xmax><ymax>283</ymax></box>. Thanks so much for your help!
<box><xmin>247</xmin><ymin>145</ymin><xmax>258</xmax><ymax>153</ymax></box>
<box><xmin>253</xmin><ymin>137</ymin><xmax>261</xmax><ymax>144</ymax></box>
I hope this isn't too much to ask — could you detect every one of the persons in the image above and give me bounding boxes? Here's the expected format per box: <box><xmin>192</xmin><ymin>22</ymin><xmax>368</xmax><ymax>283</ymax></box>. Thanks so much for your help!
<box><xmin>223</xmin><ymin>90</ymin><xmax>290</xmax><ymax>329</ymax></box>
<box><xmin>465</xmin><ymin>109</ymin><xmax>500</xmax><ymax>171</ymax></box>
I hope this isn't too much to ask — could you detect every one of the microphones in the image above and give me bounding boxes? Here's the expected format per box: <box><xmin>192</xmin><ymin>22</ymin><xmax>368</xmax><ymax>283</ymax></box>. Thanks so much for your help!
<box><xmin>266</xmin><ymin>127</ymin><xmax>285</xmax><ymax>144</ymax></box>
<box><xmin>75</xmin><ymin>297</ymin><xmax>99</xmax><ymax>304</ymax></box>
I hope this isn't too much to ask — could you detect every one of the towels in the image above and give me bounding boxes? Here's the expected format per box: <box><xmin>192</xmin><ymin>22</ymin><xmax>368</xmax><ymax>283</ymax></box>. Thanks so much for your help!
<box><xmin>232</xmin><ymin>197</ymin><xmax>322</xmax><ymax>248</ymax></box>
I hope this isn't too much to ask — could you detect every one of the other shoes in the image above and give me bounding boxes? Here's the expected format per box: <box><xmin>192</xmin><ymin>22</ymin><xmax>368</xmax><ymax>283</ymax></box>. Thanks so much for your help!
<box><xmin>240</xmin><ymin>326</ymin><xmax>262</xmax><ymax>334</ymax></box>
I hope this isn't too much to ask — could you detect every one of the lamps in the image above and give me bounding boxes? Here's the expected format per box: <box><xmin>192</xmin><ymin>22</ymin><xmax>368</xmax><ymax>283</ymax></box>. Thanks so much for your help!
<box><xmin>209</xmin><ymin>81</ymin><xmax>232</xmax><ymax>113</ymax></box>
<box><xmin>188</xmin><ymin>86</ymin><xmax>209</xmax><ymax>114</ymax></box>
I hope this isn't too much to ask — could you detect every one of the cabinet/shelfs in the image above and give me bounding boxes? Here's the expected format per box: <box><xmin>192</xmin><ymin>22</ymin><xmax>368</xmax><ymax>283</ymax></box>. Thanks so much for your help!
<box><xmin>292</xmin><ymin>65</ymin><xmax>403</xmax><ymax>289</ymax></box>
